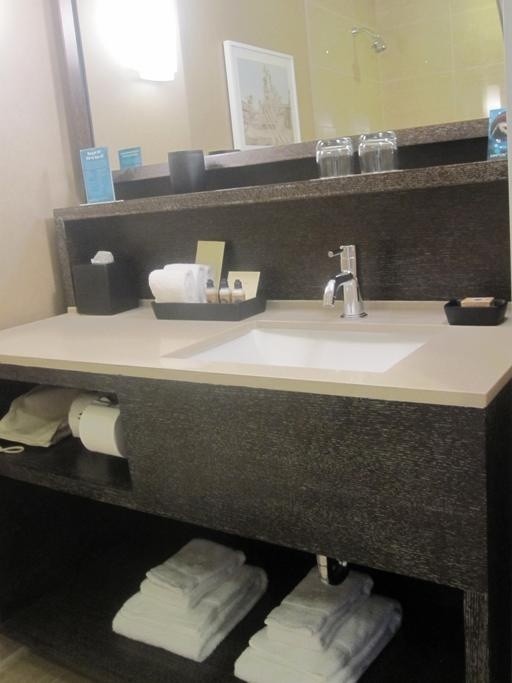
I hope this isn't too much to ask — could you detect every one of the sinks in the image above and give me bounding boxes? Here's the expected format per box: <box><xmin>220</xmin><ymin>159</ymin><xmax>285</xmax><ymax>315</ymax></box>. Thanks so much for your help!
<box><xmin>161</xmin><ymin>318</ymin><xmax>434</xmax><ymax>377</ymax></box>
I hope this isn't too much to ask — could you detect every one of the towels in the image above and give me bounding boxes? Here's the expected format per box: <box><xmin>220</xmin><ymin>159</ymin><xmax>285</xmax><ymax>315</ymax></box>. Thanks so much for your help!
<box><xmin>148</xmin><ymin>262</ymin><xmax>210</xmax><ymax>302</ymax></box>
<box><xmin>232</xmin><ymin>560</ymin><xmax>402</xmax><ymax>683</ymax></box>
<box><xmin>112</xmin><ymin>539</ymin><xmax>269</xmax><ymax>664</ymax></box>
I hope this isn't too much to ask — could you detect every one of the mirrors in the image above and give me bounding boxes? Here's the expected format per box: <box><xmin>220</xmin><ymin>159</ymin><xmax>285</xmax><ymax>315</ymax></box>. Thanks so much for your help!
<box><xmin>50</xmin><ymin>1</ymin><xmax>508</xmax><ymax>203</ymax></box>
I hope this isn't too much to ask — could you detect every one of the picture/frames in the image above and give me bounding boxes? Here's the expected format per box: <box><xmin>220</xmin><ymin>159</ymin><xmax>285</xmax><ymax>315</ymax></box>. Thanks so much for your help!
<box><xmin>223</xmin><ymin>41</ymin><xmax>302</xmax><ymax>151</ymax></box>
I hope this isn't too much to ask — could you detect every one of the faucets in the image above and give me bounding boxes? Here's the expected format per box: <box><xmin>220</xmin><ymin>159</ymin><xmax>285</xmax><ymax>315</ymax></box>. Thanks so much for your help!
<box><xmin>324</xmin><ymin>244</ymin><xmax>369</xmax><ymax>319</ymax></box>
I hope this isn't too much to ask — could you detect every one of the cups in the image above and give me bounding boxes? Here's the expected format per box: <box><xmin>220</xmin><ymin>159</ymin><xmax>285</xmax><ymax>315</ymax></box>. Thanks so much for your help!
<box><xmin>166</xmin><ymin>150</ymin><xmax>205</xmax><ymax>194</ymax></box>
<box><xmin>315</xmin><ymin>136</ymin><xmax>355</xmax><ymax>178</ymax></box>
<box><xmin>357</xmin><ymin>130</ymin><xmax>399</xmax><ymax>173</ymax></box>
